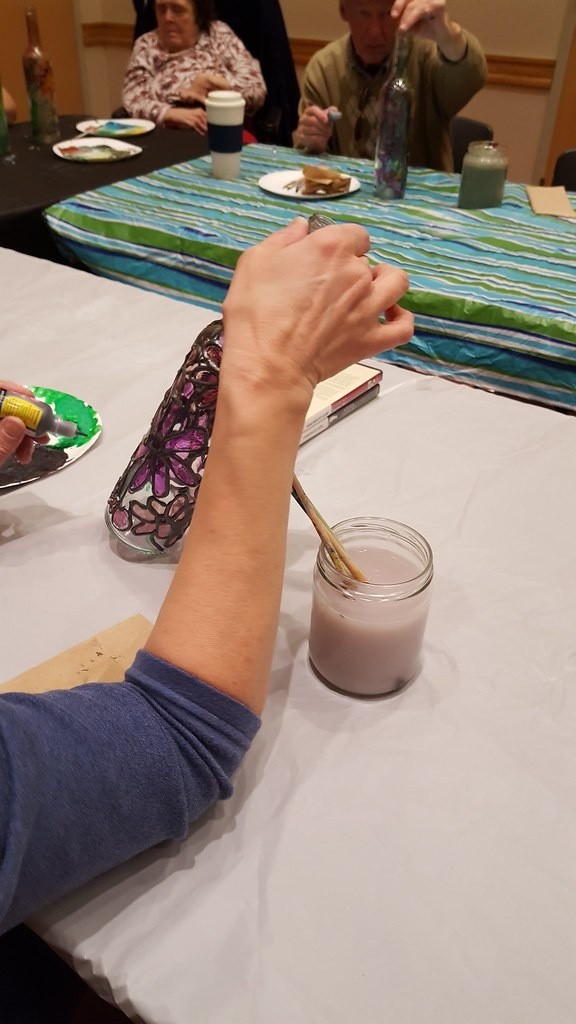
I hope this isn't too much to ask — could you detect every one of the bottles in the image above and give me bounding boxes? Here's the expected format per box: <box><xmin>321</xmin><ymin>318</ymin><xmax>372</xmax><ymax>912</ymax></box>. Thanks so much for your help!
<box><xmin>457</xmin><ymin>141</ymin><xmax>508</xmax><ymax>210</ymax></box>
<box><xmin>104</xmin><ymin>316</ymin><xmax>225</xmax><ymax>555</ymax></box>
<box><xmin>372</xmin><ymin>33</ymin><xmax>414</xmax><ymax>199</ymax></box>
<box><xmin>21</xmin><ymin>5</ymin><xmax>60</xmax><ymax>143</ymax></box>
<box><xmin>308</xmin><ymin>515</ymin><xmax>435</xmax><ymax>697</ymax></box>
<box><xmin>0</xmin><ymin>386</ymin><xmax>88</xmax><ymax>437</ymax></box>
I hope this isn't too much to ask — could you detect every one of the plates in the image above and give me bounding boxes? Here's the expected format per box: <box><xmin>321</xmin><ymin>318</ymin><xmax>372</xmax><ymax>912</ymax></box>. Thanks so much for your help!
<box><xmin>258</xmin><ymin>169</ymin><xmax>361</xmax><ymax>199</ymax></box>
<box><xmin>0</xmin><ymin>383</ymin><xmax>102</xmax><ymax>490</ymax></box>
<box><xmin>52</xmin><ymin>137</ymin><xmax>143</xmax><ymax>161</ymax></box>
<box><xmin>76</xmin><ymin>119</ymin><xmax>156</xmax><ymax>135</ymax></box>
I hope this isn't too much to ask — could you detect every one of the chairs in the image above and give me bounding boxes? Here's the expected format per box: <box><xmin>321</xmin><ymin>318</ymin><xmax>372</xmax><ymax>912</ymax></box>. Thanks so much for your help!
<box><xmin>450</xmin><ymin>115</ymin><xmax>495</xmax><ymax>173</ymax></box>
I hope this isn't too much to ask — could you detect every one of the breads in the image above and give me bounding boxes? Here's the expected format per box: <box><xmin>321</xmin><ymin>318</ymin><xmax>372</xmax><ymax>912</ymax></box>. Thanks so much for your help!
<box><xmin>300</xmin><ymin>164</ymin><xmax>351</xmax><ymax>195</ymax></box>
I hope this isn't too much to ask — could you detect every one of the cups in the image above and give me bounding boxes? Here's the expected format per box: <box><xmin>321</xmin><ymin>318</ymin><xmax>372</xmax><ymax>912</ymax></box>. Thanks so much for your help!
<box><xmin>204</xmin><ymin>91</ymin><xmax>246</xmax><ymax>178</ymax></box>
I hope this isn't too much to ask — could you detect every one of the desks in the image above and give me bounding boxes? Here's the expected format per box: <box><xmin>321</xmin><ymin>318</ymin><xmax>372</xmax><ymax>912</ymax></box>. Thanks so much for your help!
<box><xmin>60</xmin><ymin>144</ymin><xmax>576</xmax><ymax>416</ymax></box>
<box><xmin>0</xmin><ymin>242</ymin><xmax>576</xmax><ymax>1024</ymax></box>
<box><xmin>0</xmin><ymin>112</ymin><xmax>212</xmax><ymax>239</ymax></box>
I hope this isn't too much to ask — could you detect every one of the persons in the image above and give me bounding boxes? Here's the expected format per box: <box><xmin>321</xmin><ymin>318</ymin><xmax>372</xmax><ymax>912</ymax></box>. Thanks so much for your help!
<box><xmin>0</xmin><ymin>217</ymin><xmax>418</xmax><ymax>939</ymax></box>
<box><xmin>298</xmin><ymin>0</ymin><xmax>488</xmax><ymax>174</ymax></box>
<box><xmin>121</xmin><ymin>0</ymin><xmax>267</xmax><ymax>138</ymax></box>
